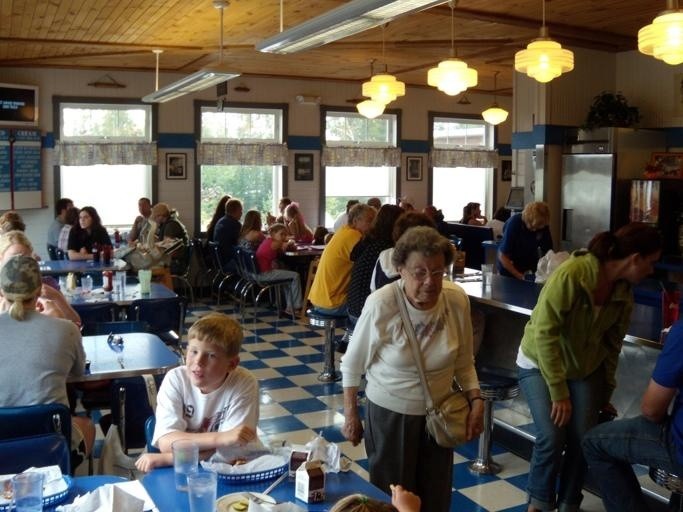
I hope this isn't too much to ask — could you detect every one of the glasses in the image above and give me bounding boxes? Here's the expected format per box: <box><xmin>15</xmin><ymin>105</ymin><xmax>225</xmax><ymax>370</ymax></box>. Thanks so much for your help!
<box><xmin>398</xmin><ymin>265</ymin><xmax>451</xmax><ymax>282</ymax></box>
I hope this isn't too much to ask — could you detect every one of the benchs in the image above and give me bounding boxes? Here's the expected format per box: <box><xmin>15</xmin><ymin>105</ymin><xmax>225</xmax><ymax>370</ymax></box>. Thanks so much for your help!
<box><xmin>446</xmin><ymin>221</ymin><xmax>495</xmax><ymax>269</ymax></box>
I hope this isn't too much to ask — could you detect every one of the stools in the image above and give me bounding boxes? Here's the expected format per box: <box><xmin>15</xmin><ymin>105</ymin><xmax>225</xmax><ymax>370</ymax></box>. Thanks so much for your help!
<box><xmin>303</xmin><ymin>307</ymin><xmax>351</xmax><ymax>383</ymax></box>
<box><xmin>467</xmin><ymin>370</ymin><xmax>522</xmax><ymax>477</ymax></box>
<box><xmin>648</xmin><ymin>464</ymin><xmax>683</xmax><ymax>512</ymax></box>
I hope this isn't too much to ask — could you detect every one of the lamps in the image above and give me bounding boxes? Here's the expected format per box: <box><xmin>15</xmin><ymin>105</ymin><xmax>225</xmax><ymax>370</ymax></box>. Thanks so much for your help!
<box><xmin>139</xmin><ymin>0</ymin><xmax>243</xmax><ymax>104</ymax></box>
<box><xmin>482</xmin><ymin>70</ymin><xmax>509</xmax><ymax>126</ymax></box>
<box><xmin>255</xmin><ymin>0</ymin><xmax>444</xmax><ymax>57</ymax></box>
<box><xmin>514</xmin><ymin>0</ymin><xmax>575</xmax><ymax>83</ymax></box>
<box><xmin>356</xmin><ymin>99</ymin><xmax>386</xmax><ymax>119</ymax></box>
<box><xmin>362</xmin><ymin>22</ymin><xmax>405</xmax><ymax>105</ymax></box>
<box><xmin>427</xmin><ymin>0</ymin><xmax>478</xmax><ymax>97</ymax></box>
<box><xmin>636</xmin><ymin>0</ymin><xmax>683</xmax><ymax>66</ymax></box>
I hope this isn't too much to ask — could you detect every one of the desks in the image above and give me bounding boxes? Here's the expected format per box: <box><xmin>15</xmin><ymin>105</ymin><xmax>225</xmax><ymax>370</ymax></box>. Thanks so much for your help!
<box><xmin>66</xmin><ymin>331</ymin><xmax>180</xmax><ymax>420</ymax></box>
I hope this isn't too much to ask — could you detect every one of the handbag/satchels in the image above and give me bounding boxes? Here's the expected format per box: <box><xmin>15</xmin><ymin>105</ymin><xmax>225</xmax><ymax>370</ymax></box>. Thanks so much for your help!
<box><xmin>425</xmin><ymin>391</ymin><xmax>472</xmax><ymax>448</ymax></box>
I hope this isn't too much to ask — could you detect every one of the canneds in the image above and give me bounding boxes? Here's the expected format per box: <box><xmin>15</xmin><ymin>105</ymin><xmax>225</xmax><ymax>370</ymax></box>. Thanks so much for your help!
<box><xmin>102</xmin><ymin>269</ymin><xmax>113</xmax><ymax>291</ymax></box>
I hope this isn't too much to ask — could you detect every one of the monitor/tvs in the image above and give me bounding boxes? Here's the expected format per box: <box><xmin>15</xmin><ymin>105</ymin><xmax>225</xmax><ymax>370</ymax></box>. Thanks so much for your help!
<box><xmin>504</xmin><ymin>187</ymin><xmax>525</xmax><ymax>210</ymax></box>
<box><xmin>0</xmin><ymin>83</ymin><xmax>40</xmax><ymax>128</ymax></box>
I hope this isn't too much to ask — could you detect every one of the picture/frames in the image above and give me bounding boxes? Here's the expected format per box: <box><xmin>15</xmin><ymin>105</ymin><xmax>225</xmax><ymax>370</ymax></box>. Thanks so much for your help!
<box><xmin>501</xmin><ymin>159</ymin><xmax>512</xmax><ymax>182</ymax></box>
<box><xmin>406</xmin><ymin>155</ymin><xmax>423</xmax><ymax>181</ymax></box>
<box><xmin>165</xmin><ymin>152</ymin><xmax>187</xmax><ymax>180</ymax></box>
<box><xmin>294</xmin><ymin>152</ymin><xmax>314</xmax><ymax>181</ymax></box>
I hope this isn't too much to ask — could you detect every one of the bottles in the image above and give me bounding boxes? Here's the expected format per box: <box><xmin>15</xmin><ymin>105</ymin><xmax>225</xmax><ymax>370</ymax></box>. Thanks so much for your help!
<box><xmin>101</xmin><ymin>270</ymin><xmax>114</xmax><ymax>291</ymax></box>
<box><xmin>628</xmin><ymin>180</ymin><xmax>660</xmax><ymax>225</ymax></box>
<box><xmin>445</xmin><ymin>235</ymin><xmax>466</xmax><ymax>279</ymax></box>
<box><xmin>114</xmin><ymin>230</ymin><xmax>120</xmax><ymax>241</ymax></box>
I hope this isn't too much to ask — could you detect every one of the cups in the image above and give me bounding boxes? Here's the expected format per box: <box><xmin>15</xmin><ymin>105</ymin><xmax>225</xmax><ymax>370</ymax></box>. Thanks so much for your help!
<box><xmin>173</xmin><ymin>439</ymin><xmax>218</xmax><ymax>512</ymax></box>
<box><xmin>481</xmin><ymin>264</ymin><xmax>494</xmax><ymax>286</ymax></box>
<box><xmin>138</xmin><ymin>269</ymin><xmax>152</xmax><ymax>294</ymax></box>
<box><xmin>661</xmin><ymin>290</ymin><xmax>678</xmax><ymax>326</ymax></box>
<box><xmin>116</xmin><ymin>271</ymin><xmax>127</xmax><ymax>293</ymax></box>
<box><xmin>92</xmin><ymin>243</ymin><xmax>111</xmax><ymax>265</ymax></box>
<box><xmin>659</xmin><ymin>328</ymin><xmax>671</xmax><ymax>343</ymax></box>
<box><xmin>58</xmin><ymin>273</ymin><xmax>93</xmax><ymax>296</ymax></box>
<box><xmin>12</xmin><ymin>472</ymin><xmax>46</xmax><ymax>511</ymax></box>
<box><xmin>122</xmin><ymin>232</ymin><xmax>127</xmax><ymax>241</ymax></box>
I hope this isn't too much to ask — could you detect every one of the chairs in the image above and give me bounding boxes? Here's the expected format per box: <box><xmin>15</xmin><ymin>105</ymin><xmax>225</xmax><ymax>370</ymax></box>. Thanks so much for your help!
<box><xmin>0</xmin><ymin>433</ymin><xmax>74</xmax><ymax>476</ymax></box>
<box><xmin>93</xmin><ymin>320</ymin><xmax>185</xmax><ymax>454</ymax></box>
<box><xmin>1</xmin><ymin>402</ymin><xmax>94</xmax><ymax>477</ymax></box>
<box><xmin>143</xmin><ymin>413</ymin><xmax>161</xmax><ymax>453</ymax></box>
<box><xmin>34</xmin><ymin>211</ymin><xmax>327</xmax><ymax>328</ymax></box>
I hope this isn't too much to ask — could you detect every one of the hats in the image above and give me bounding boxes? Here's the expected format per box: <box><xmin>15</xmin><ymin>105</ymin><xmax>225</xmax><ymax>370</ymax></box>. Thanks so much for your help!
<box><xmin>0</xmin><ymin>255</ymin><xmax>43</xmax><ymax>294</ymax></box>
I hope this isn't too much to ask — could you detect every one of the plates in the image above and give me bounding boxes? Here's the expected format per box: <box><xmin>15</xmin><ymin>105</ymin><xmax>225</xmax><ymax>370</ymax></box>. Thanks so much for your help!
<box><xmin>216</xmin><ymin>489</ymin><xmax>276</xmax><ymax>511</ymax></box>
